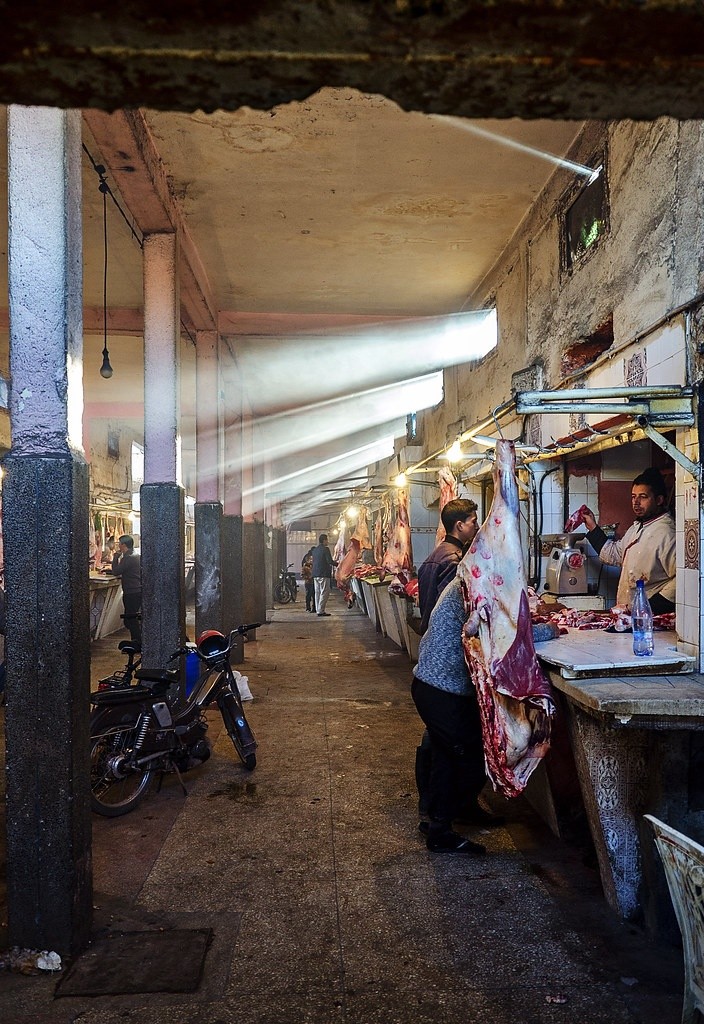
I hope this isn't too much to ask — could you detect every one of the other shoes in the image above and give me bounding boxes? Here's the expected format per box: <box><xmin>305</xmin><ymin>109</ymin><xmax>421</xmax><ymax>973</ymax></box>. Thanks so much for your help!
<box><xmin>318</xmin><ymin>612</ymin><xmax>331</xmax><ymax>616</ymax></box>
<box><xmin>348</xmin><ymin>592</ymin><xmax>356</xmax><ymax>609</ymax></box>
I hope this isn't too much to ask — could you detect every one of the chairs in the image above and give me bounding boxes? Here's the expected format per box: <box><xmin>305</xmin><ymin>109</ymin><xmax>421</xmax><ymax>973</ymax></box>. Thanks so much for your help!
<box><xmin>643</xmin><ymin>814</ymin><xmax>704</xmax><ymax>1024</ymax></box>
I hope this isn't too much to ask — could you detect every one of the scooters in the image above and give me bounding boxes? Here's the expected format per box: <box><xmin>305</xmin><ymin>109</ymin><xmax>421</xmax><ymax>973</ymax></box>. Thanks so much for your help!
<box><xmin>91</xmin><ymin>622</ymin><xmax>259</xmax><ymax>817</ymax></box>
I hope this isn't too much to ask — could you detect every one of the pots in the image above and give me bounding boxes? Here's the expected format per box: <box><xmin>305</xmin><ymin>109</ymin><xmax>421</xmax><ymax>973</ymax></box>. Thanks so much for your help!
<box><xmin>601</xmin><ymin>521</ymin><xmax>620</xmax><ymax>530</ymax></box>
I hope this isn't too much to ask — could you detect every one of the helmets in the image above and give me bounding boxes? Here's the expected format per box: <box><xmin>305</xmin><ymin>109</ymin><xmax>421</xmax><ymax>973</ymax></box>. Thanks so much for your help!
<box><xmin>198</xmin><ymin>630</ymin><xmax>227</xmax><ymax>655</ymax></box>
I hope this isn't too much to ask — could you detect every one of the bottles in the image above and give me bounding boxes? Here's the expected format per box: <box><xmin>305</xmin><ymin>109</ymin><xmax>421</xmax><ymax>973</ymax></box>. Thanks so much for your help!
<box><xmin>631</xmin><ymin>579</ymin><xmax>654</xmax><ymax>656</ymax></box>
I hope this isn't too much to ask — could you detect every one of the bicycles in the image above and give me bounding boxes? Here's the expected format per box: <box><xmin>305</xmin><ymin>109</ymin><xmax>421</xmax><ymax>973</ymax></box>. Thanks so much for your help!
<box><xmin>94</xmin><ymin>638</ymin><xmax>146</xmax><ymax>694</ymax></box>
<box><xmin>272</xmin><ymin>562</ymin><xmax>300</xmax><ymax>606</ymax></box>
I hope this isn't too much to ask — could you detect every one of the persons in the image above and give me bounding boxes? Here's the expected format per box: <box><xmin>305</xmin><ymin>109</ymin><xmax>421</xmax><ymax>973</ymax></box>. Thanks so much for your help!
<box><xmin>580</xmin><ymin>469</ymin><xmax>676</xmax><ymax>616</ymax></box>
<box><xmin>300</xmin><ymin>554</ymin><xmax>317</xmax><ymax>613</ymax></box>
<box><xmin>312</xmin><ymin>534</ymin><xmax>337</xmax><ymax>616</ymax></box>
<box><xmin>0</xmin><ymin>569</ymin><xmax>5</xmax><ymax>694</ymax></box>
<box><xmin>101</xmin><ymin>536</ymin><xmax>115</xmax><ymax>563</ymax></box>
<box><xmin>361</xmin><ymin>537</ymin><xmax>375</xmax><ymax>564</ymax></box>
<box><xmin>407</xmin><ymin>574</ymin><xmax>560</xmax><ymax>854</ymax></box>
<box><xmin>417</xmin><ymin>499</ymin><xmax>504</xmax><ymax>827</ymax></box>
<box><xmin>301</xmin><ymin>546</ymin><xmax>316</xmax><ymax>567</ymax></box>
<box><xmin>99</xmin><ymin>535</ymin><xmax>141</xmax><ymax>641</ymax></box>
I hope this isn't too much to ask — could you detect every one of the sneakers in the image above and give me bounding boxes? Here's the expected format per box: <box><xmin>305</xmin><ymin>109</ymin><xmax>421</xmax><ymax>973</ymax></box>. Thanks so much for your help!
<box><xmin>426</xmin><ymin>822</ymin><xmax>486</xmax><ymax>855</ymax></box>
<box><xmin>454</xmin><ymin>799</ymin><xmax>506</xmax><ymax>827</ymax></box>
